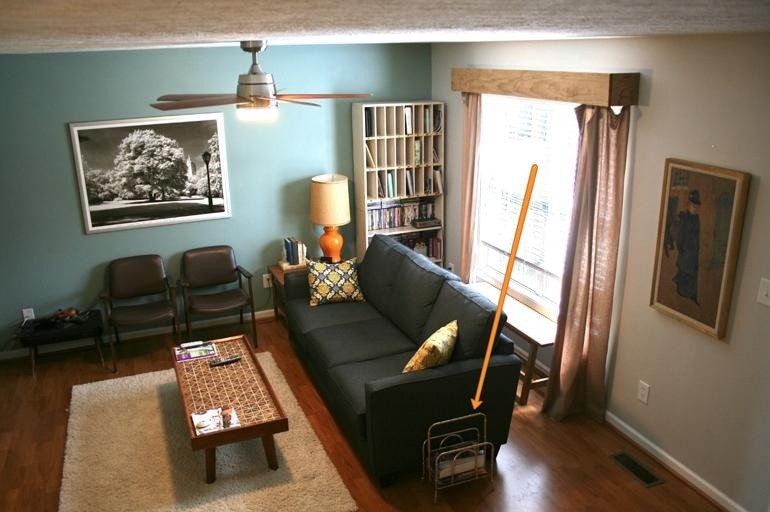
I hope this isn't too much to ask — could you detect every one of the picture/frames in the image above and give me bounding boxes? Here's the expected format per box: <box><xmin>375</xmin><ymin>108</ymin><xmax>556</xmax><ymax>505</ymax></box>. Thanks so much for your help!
<box><xmin>648</xmin><ymin>155</ymin><xmax>750</xmax><ymax>342</ymax></box>
<box><xmin>67</xmin><ymin>110</ymin><xmax>234</xmax><ymax>235</ymax></box>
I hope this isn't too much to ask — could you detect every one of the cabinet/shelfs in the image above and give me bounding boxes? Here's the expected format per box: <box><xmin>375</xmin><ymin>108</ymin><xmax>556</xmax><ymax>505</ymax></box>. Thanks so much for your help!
<box><xmin>351</xmin><ymin>99</ymin><xmax>446</xmax><ymax>270</ymax></box>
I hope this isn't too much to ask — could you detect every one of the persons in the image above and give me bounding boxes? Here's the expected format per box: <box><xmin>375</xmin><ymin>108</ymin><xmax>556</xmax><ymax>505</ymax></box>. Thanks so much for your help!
<box><xmin>669</xmin><ymin>188</ymin><xmax>703</xmax><ymax>307</ymax></box>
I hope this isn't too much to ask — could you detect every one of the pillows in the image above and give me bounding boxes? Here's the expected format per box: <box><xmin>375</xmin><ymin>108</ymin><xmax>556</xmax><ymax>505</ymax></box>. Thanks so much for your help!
<box><xmin>401</xmin><ymin>319</ymin><xmax>460</xmax><ymax>374</ymax></box>
<box><xmin>305</xmin><ymin>256</ymin><xmax>368</xmax><ymax>308</ymax></box>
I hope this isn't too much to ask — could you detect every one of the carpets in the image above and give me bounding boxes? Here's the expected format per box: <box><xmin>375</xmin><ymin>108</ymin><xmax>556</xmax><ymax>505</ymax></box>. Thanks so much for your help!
<box><xmin>55</xmin><ymin>349</ymin><xmax>363</xmax><ymax>512</ymax></box>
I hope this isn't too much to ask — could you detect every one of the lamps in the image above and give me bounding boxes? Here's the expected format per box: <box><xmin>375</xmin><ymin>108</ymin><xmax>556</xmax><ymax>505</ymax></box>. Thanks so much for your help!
<box><xmin>309</xmin><ymin>171</ymin><xmax>352</xmax><ymax>262</ymax></box>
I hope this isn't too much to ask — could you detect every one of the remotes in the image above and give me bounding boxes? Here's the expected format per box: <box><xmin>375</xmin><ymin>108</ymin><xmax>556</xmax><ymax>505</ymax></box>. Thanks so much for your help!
<box><xmin>210</xmin><ymin>355</ymin><xmax>241</xmax><ymax>366</ymax></box>
<box><xmin>181</xmin><ymin>341</ymin><xmax>203</xmax><ymax>348</ymax></box>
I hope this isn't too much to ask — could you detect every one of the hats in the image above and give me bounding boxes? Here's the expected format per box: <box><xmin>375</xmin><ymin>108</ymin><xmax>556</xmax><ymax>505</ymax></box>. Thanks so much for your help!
<box><xmin>687</xmin><ymin>188</ymin><xmax>701</xmax><ymax>205</ymax></box>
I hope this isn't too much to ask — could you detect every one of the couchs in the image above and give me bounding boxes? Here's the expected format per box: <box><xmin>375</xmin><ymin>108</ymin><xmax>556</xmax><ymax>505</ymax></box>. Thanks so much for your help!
<box><xmin>284</xmin><ymin>231</ymin><xmax>521</xmax><ymax>489</ymax></box>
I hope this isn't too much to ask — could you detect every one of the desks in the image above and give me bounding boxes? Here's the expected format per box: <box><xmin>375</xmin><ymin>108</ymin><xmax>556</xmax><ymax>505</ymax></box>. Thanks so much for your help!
<box><xmin>18</xmin><ymin>305</ymin><xmax>108</xmax><ymax>380</ymax></box>
<box><xmin>466</xmin><ymin>279</ymin><xmax>559</xmax><ymax>410</ymax></box>
<box><xmin>266</xmin><ymin>258</ymin><xmax>315</xmax><ymax>320</ymax></box>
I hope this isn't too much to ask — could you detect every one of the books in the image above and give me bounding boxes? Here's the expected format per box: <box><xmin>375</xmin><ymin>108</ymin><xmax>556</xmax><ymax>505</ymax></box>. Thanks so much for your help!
<box><xmin>180</xmin><ymin>341</ymin><xmax>216</xmax><ymax>357</ymax></box>
<box><xmin>430</xmin><ymin>441</ymin><xmax>486</xmax><ymax>486</ymax></box>
<box><xmin>366</xmin><ymin>104</ymin><xmax>445</xmax><ymax>264</ymax></box>
<box><xmin>283</xmin><ymin>236</ymin><xmax>308</xmax><ymax>266</ymax></box>
<box><xmin>190</xmin><ymin>405</ymin><xmax>241</xmax><ymax>438</ymax></box>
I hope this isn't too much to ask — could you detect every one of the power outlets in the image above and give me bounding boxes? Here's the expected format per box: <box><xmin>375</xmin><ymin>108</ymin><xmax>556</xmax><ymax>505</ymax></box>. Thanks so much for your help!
<box><xmin>262</xmin><ymin>274</ymin><xmax>273</xmax><ymax>289</ymax></box>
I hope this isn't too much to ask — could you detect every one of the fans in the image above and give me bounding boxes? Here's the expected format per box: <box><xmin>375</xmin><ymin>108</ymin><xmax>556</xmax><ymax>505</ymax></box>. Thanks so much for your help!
<box><xmin>150</xmin><ymin>38</ymin><xmax>376</xmax><ymax>122</ymax></box>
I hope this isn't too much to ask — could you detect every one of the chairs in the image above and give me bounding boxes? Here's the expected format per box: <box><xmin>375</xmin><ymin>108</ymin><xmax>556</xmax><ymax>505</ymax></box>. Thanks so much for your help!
<box><xmin>100</xmin><ymin>254</ymin><xmax>183</xmax><ymax>371</ymax></box>
<box><xmin>177</xmin><ymin>243</ymin><xmax>259</xmax><ymax>350</ymax></box>
